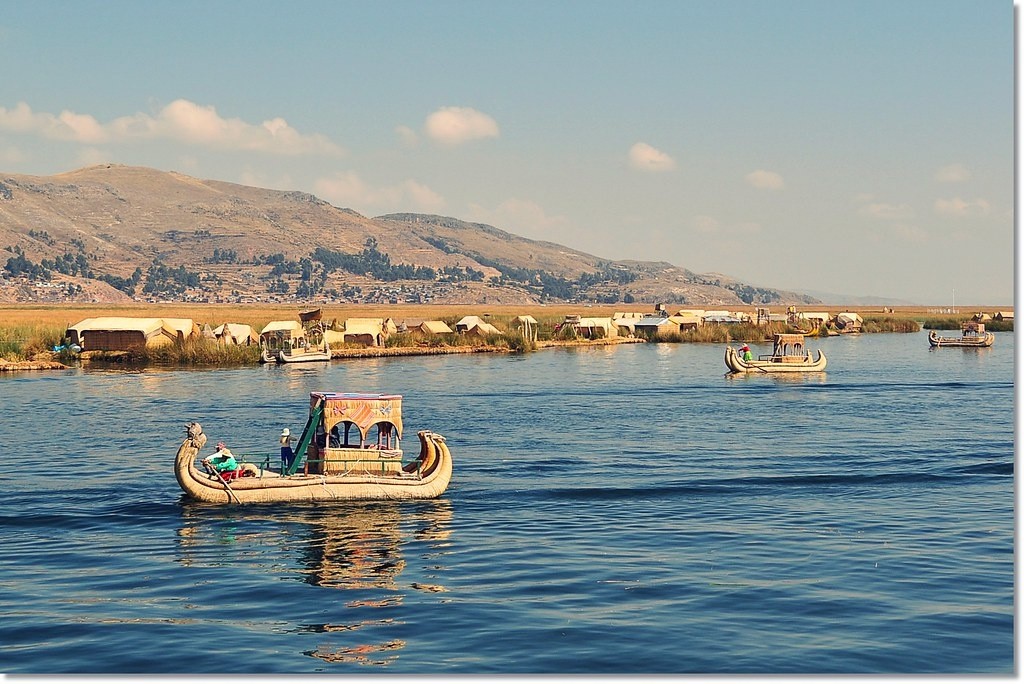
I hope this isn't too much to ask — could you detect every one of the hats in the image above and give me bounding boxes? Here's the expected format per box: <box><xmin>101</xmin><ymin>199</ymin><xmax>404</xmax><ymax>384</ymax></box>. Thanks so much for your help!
<box><xmin>220</xmin><ymin>448</ymin><xmax>231</xmax><ymax>458</ymax></box>
<box><xmin>281</xmin><ymin>428</ymin><xmax>291</xmax><ymax>436</ymax></box>
<box><xmin>742</xmin><ymin>343</ymin><xmax>748</xmax><ymax>348</ymax></box>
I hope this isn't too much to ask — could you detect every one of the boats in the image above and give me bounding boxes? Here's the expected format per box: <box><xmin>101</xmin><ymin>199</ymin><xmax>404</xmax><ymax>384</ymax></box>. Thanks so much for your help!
<box><xmin>174</xmin><ymin>391</ymin><xmax>453</xmax><ymax>503</ymax></box>
<box><xmin>724</xmin><ymin>333</ymin><xmax>826</xmax><ymax>374</ymax></box>
<box><xmin>928</xmin><ymin>321</ymin><xmax>994</xmax><ymax>349</ymax></box>
<box><xmin>785</xmin><ymin>320</ymin><xmax>820</xmax><ymax>337</ymax></box>
<box><xmin>259</xmin><ymin>327</ymin><xmax>331</xmax><ymax>362</ymax></box>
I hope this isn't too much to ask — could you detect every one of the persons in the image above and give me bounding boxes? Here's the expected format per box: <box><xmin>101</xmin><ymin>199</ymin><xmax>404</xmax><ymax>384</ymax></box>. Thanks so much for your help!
<box><xmin>738</xmin><ymin>343</ymin><xmax>753</xmax><ymax>363</ymax></box>
<box><xmin>200</xmin><ymin>441</ymin><xmax>243</xmax><ymax>481</ymax></box>
<box><xmin>331</xmin><ymin>426</ymin><xmax>340</xmax><ymax>443</ymax></box>
<box><xmin>280</xmin><ymin>428</ymin><xmax>297</xmax><ymax>470</ymax></box>
